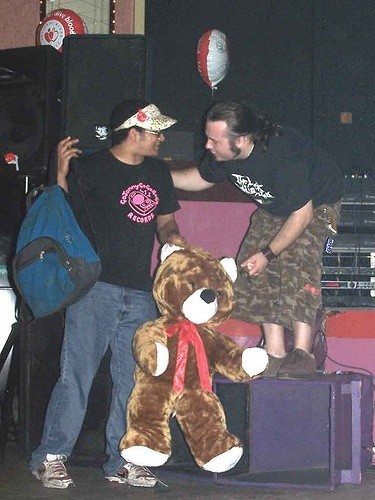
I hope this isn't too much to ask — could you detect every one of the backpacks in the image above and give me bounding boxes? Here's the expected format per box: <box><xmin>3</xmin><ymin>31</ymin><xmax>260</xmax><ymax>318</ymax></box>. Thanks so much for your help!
<box><xmin>11</xmin><ymin>184</ymin><xmax>102</xmax><ymax>324</ymax></box>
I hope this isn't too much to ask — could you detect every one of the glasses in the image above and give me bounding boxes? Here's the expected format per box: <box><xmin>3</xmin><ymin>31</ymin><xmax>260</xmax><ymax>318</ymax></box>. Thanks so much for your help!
<box><xmin>139</xmin><ymin>129</ymin><xmax>162</xmax><ymax>135</ymax></box>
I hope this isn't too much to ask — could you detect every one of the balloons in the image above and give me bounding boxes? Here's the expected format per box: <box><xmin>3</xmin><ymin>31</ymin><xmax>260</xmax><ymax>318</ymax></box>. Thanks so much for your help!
<box><xmin>196</xmin><ymin>29</ymin><xmax>231</xmax><ymax>98</ymax></box>
<box><xmin>35</xmin><ymin>8</ymin><xmax>88</xmax><ymax>55</ymax></box>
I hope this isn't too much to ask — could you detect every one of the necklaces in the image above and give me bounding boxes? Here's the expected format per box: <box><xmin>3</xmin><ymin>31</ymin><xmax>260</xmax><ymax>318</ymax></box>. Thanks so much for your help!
<box><xmin>245</xmin><ymin>145</ymin><xmax>255</xmax><ymax>158</ymax></box>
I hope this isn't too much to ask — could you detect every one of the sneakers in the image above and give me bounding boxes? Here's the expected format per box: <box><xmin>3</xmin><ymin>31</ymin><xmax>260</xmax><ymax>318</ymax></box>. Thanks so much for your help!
<box><xmin>104</xmin><ymin>462</ymin><xmax>157</xmax><ymax>487</ymax></box>
<box><xmin>30</xmin><ymin>454</ymin><xmax>75</xmax><ymax>489</ymax></box>
<box><xmin>259</xmin><ymin>354</ymin><xmax>285</xmax><ymax>379</ymax></box>
<box><xmin>276</xmin><ymin>349</ymin><xmax>317</xmax><ymax>379</ymax></box>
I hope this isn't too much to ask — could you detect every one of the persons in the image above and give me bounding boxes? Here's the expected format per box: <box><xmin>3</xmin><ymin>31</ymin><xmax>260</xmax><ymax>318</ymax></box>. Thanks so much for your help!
<box><xmin>169</xmin><ymin>100</ymin><xmax>344</xmax><ymax>381</ymax></box>
<box><xmin>29</xmin><ymin>99</ymin><xmax>177</xmax><ymax>490</ymax></box>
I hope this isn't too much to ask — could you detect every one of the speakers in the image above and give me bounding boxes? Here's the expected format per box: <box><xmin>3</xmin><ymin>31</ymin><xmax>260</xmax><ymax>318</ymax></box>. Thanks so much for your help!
<box><xmin>0</xmin><ymin>45</ymin><xmax>62</xmax><ymax>180</ymax></box>
<box><xmin>16</xmin><ymin>294</ymin><xmax>111</xmax><ymax>464</ymax></box>
<box><xmin>62</xmin><ymin>34</ymin><xmax>154</xmax><ymax>152</ymax></box>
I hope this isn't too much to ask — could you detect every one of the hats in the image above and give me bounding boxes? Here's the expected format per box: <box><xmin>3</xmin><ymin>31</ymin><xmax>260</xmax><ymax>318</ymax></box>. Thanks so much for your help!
<box><xmin>113</xmin><ymin>103</ymin><xmax>178</xmax><ymax>131</ymax></box>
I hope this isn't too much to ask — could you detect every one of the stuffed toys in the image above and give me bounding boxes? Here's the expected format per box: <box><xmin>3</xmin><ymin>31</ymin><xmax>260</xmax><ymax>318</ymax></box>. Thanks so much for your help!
<box><xmin>118</xmin><ymin>242</ymin><xmax>269</xmax><ymax>474</ymax></box>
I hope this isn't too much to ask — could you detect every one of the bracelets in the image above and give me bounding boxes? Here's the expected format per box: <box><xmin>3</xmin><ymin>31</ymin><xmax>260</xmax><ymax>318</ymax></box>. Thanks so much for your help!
<box><xmin>261</xmin><ymin>246</ymin><xmax>276</xmax><ymax>263</ymax></box>
